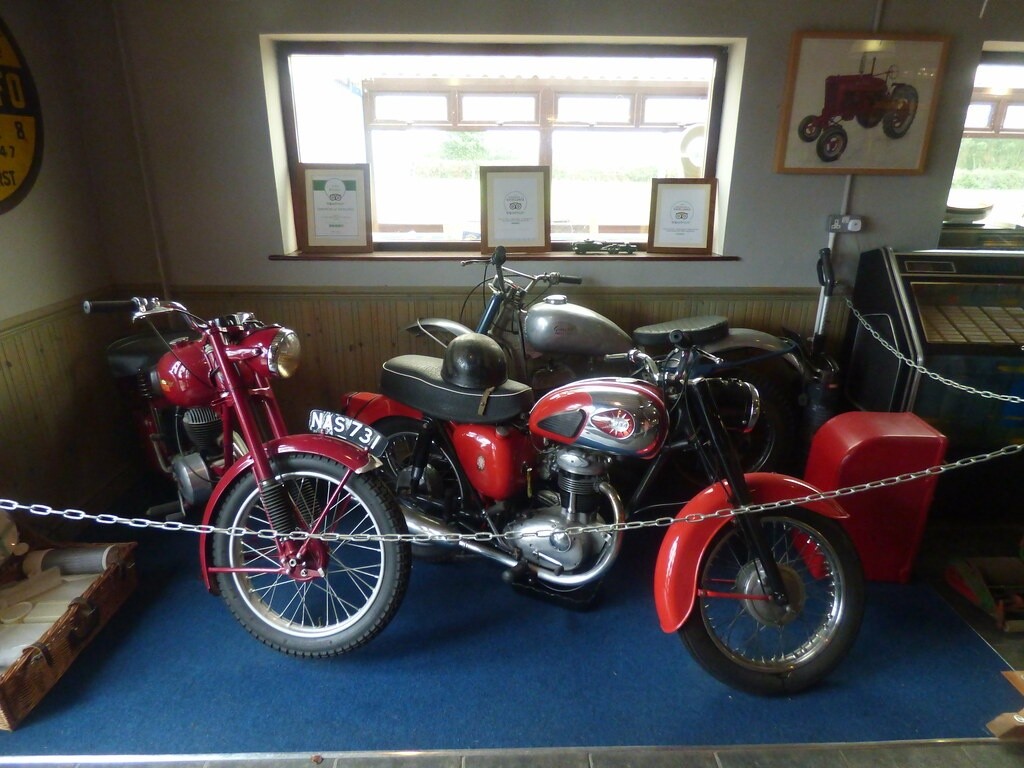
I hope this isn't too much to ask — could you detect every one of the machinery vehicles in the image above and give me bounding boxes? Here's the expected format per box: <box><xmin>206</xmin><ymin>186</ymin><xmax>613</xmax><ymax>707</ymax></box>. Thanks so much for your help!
<box><xmin>798</xmin><ymin>52</ymin><xmax>919</xmax><ymax>163</ymax></box>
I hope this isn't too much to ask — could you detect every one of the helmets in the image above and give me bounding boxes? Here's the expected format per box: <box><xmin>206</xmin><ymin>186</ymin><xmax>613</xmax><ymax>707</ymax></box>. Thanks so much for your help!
<box><xmin>441</xmin><ymin>333</ymin><xmax>508</xmax><ymax>389</ymax></box>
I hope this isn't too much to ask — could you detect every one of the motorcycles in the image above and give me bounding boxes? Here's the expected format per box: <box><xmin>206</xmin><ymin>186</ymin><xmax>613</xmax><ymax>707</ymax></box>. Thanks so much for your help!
<box><xmin>333</xmin><ymin>329</ymin><xmax>867</xmax><ymax>697</ymax></box>
<box><xmin>83</xmin><ymin>298</ymin><xmax>410</xmax><ymax>660</ymax></box>
<box><xmin>408</xmin><ymin>245</ymin><xmax>811</xmax><ymax>492</ymax></box>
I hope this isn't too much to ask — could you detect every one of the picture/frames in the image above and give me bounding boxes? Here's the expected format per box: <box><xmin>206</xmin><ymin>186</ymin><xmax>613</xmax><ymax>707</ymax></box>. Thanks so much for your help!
<box><xmin>648</xmin><ymin>177</ymin><xmax>718</xmax><ymax>257</ymax></box>
<box><xmin>776</xmin><ymin>30</ymin><xmax>954</xmax><ymax>177</ymax></box>
<box><xmin>479</xmin><ymin>164</ymin><xmax>554</xmax><ymax>254</ymax></box>
<box><xmin>294</xmin><ymin>160</ymin><xmax>376</xmax><ymax>259</ymax></box>
